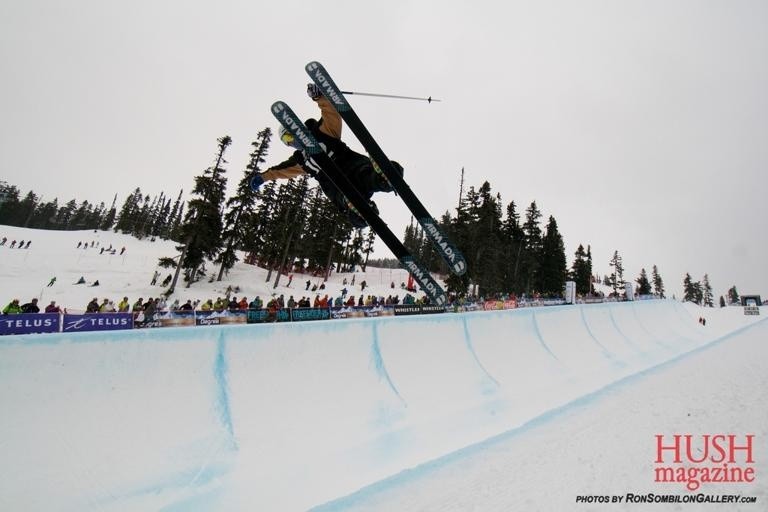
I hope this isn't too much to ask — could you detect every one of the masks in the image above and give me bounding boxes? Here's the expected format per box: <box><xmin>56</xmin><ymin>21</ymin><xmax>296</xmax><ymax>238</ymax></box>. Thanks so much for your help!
<box><xmin>281</xmin><ymin>134</ymin><xmax>296</xmax><ymax>147</ymax></box>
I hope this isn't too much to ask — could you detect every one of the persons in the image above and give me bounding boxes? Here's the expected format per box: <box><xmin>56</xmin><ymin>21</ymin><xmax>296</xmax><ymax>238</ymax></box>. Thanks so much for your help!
<box><xmin>244</xmin><ymin>83</ymin><xmax>403</xmax><ymax>225</ymax></box>
<box><xmin>698</xmin><ymin>317</ymin><xmax>706</xmax><ymax>326</ymax></box>
<box><xmin>1</xmin><ymin>223</ymin><xmax>663</xmax><ymax>316</ymax></box>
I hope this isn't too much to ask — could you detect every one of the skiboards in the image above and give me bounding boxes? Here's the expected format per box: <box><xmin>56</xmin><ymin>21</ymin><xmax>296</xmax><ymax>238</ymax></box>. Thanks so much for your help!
<box><xmin>271</xmin><ymin>62</ymin><xmax>468</xmax><ymax>308</ymax></box>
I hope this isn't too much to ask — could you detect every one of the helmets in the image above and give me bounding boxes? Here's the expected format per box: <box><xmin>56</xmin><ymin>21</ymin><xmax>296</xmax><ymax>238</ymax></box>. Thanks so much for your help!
<box><xmin>277</xmin><ymin>122</ymin><xmax>290</xmax><ymax>141</ymax></box>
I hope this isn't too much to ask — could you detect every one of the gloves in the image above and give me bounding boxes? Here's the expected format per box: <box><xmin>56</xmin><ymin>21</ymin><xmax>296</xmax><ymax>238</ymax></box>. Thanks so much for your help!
<box><xmin>250</xmin><ymin>173</ymin><xmax>265</xmax><ymax>191</ymax></box>
<box><xmin>306</xmin><ymin>82</ymin><xmax>324</xmax><ymax>99</ymax></box>
